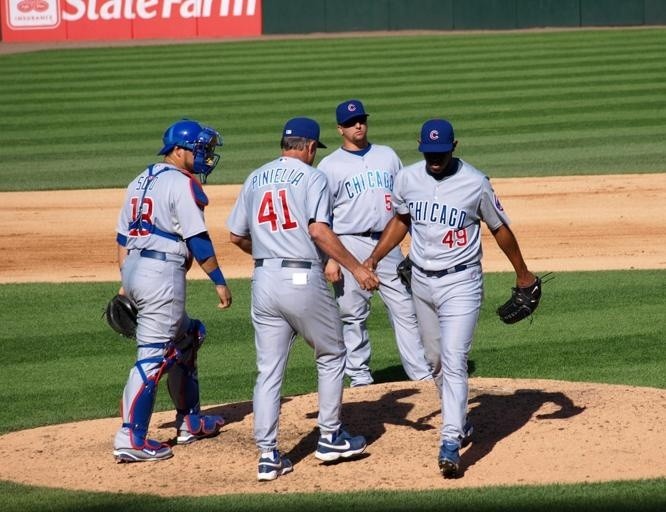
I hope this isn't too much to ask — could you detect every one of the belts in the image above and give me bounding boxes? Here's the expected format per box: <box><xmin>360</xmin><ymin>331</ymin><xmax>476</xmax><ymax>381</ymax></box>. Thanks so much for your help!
<box><xmin>127</xmin><ymin>247</ymin><xmax>166</xmax><ymax>262</ymax></box>
<box><xmin>359</xmin><ymin>231</ymin><xmax>383</xmax><ymax>238</ymax></box>
<box><xmin>412</xmin><ymin>261</ymin><xmax>468</xmax><ymax>277</ymax></box>
<box><xmin>253</xmin><ymin>258</ymin><xmax>313</xmax><ymax>269</ymax></box>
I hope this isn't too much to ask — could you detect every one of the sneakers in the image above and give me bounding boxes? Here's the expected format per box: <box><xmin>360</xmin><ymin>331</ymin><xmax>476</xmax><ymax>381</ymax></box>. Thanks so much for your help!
<box><xmin>315</xmin><ymin>429</ymin><xmax>368</xmax><ymax>461</ymax></box>
<box><xmin>111</xmin><ymin>434</ymin><xmax>174</xmax><ymax>464</ymax></box>
<box><xmin>438</xmin><ymin>440</ymin><xmax>464</xmax><ymax>476</ymax></box>
<box><xmin>175</xmin><ymin>414</ymin><xmax>224</xmax><ymax>444</ymax></box>
<box><xmin>257</xmin><ymin>450</ymin><xmax>294</xmax><ymax>482</ymax></box>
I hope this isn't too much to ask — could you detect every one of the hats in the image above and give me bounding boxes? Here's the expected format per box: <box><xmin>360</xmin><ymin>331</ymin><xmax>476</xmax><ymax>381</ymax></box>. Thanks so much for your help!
<box><xmin>282</xmin><ymin>117</ymin><xmax>327</xmax><ymax>149</ymax></box>
<box><xmin>418</xmin><ymin>117</ymin><xmax>454</xmax><ymax>154</ymax></box>
<box><xmin>335</xmin><ymin>99</ymin><xmax>370</xmax><ymax>124</ymax></box>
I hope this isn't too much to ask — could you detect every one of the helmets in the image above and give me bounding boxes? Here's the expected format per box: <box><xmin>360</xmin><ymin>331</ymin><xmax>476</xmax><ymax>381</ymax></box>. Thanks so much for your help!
<box><xmin>156</xmin><ymin>118</ymin><xmax>204</xmax><ymax>157</ymax></box>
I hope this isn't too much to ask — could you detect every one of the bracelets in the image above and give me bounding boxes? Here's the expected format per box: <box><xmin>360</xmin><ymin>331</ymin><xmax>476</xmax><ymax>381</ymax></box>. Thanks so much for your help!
<box><xmin>207</xmin><ymin>267</ymin><xmax>226</xmax><ymax>286</ymax></box>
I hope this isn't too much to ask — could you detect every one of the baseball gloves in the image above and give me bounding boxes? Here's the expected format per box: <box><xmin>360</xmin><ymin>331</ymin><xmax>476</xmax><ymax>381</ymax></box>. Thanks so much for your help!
<box><xmin>107</xmin><ymin>295</ymin><xmax>138</xmax><ymax>338</ymax></box>
<box><xmin>496</xmin><ymin>277</ymin><xmax>542</xmax><ymax>323</ymax></box>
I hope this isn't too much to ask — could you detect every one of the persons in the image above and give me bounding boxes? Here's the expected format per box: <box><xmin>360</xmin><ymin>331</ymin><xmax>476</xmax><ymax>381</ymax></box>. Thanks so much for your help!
<box><xmin>225</xmin><ymin>115</ymin><xmax>382</xmax><ymax>481</ymax></box>
<box><xmin>112</xmin><ymin>119</ymin><xmax>232</xmax><ymax>464</ymax></box>
<box><xmin>311</xmin><ymin>100</ymin><xmax>433</xmax><ymax>389</ymax></box>
<box><xmin>361</xmin><ymin>119</ymin><xmax>532</xmax><ymax>478</ymax></box>
